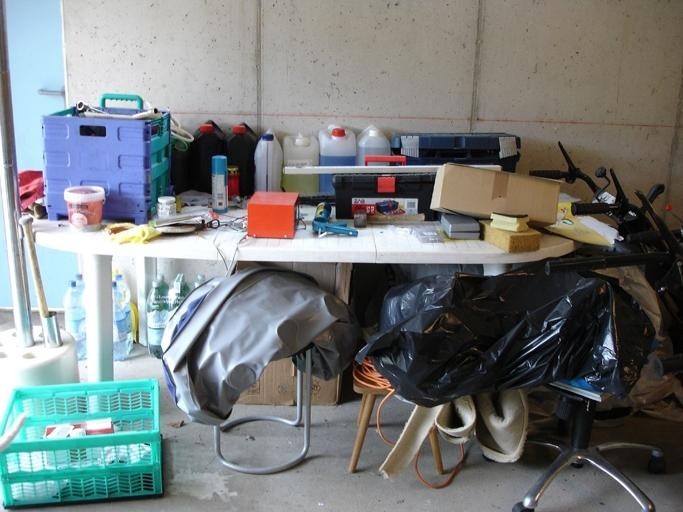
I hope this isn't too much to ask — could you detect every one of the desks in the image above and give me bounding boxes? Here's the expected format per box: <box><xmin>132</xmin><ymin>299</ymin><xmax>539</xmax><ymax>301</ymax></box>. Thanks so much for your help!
<box><xmin>18</xmin><ymin>198</ymin><xmax>579</xmax><ymax>423</ymax></box>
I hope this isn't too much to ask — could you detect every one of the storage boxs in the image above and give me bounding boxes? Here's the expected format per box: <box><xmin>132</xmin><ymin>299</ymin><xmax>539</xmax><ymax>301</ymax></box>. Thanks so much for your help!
<box><xmin>244</xmin><ymin>189</ymin><xmax>302</xmax><ymax>240</ymax></box>
<box><xmin>221</xmin><ymin>254</ymin><xmax>355</xmax><ymax>410</ymax></box>
<box><xmin>42</xmin><ymin>101</ymin><xmax>176</xmax><ymax>227</ymax></box>
<box><xmin>331</xmin><ymin>154</ymin><xmax>443</xmax><ymax>225</ymax></box>
<box><xmin>390</xmin><ymin>125</ymin><xmax>523</xmax><ymax>177</ymax></box>
<box><xmin>430</xmin><ymin>160</ymin><xmax>563</xmax><ymax>231</ymax></box>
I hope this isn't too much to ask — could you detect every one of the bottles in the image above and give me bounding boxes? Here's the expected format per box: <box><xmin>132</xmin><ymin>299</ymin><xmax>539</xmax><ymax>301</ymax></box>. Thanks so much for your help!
<box><xmin>211</xmin><ymin>155</ymin><xmax>240</xmax><ymax>214</ymax></box>
<box><xmin>64</xmin><ymin>271</ymin><xmax>206</xmax><ymax>362</ymax></box>
<box><xmin>156</xmin><ymin>194</ymin><xmax>182</xmax><ymax>221</ymax></box>
<box><xmin>353</xmin><ymin>202</ymin><xmax>366</xmax><ymax>227</ymax></box>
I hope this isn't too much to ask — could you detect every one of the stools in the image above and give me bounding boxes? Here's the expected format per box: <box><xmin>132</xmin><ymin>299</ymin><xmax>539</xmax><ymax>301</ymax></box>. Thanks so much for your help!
<box><xmin>210</xmin><ymin>331</ymin><xmax>320</xmax><ymax>477</ymax></box>
<box><xmin>340</xmin><ymin>380</ymin><xmax>446</xmax><ymax>482</ymax></box>
<box><xmin>508</xmin><ymin>376</ymin><xmax>670</xmax><ymax>512</ymax></box>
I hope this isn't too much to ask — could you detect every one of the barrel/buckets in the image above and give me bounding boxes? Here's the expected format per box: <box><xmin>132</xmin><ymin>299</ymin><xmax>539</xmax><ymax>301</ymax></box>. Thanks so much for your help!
<box><xmin>281</xmin><ymin>132</ymin><xmax>320</xmax><ymax>194</ymax></box>
<box><xmin>318</xmin><ymin>124</ymin><xmax>357</xmax><ymax>195</ymax></box>
<box><xmin>253</xmin><ymin>128</ymin><xmax>283</xmax><ymax>192</ymax></box>
<box><xmin>355</xmin><ymin>125</ymin><xmax>391</xmax><ymax>166</ymax></box>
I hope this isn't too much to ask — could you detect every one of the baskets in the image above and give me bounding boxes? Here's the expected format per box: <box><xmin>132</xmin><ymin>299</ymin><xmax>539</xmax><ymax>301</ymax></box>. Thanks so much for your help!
<box><xmin>1</xmin><ymin>378</ymin><xmax>164</xmax><ymax>508</ymax></box>
